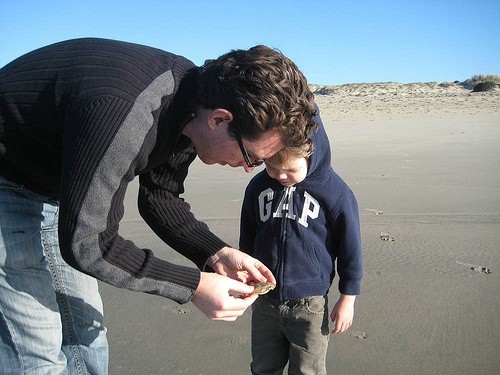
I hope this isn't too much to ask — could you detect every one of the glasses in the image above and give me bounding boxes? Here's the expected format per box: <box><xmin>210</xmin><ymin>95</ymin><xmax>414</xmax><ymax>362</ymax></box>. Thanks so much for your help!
<box><xmin>232</xmin><ymin>133</ymin><xmax>267</xmax><ymax>169</ymax></box>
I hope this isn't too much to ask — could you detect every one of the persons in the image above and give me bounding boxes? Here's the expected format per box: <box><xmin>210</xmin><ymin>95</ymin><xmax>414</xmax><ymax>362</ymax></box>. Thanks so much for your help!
<box><xmin>239</xmin><ymin>105</ymin><xmax>362</xmax><ymax>375</ymax></box>
<box><xmin>0</xmin><ymin>37</ymin><xmax>318</xmax><ymax>375</ymax></box>
